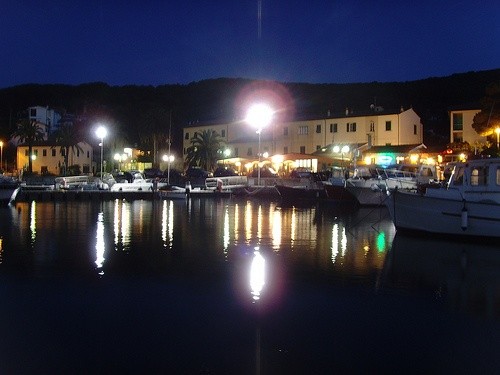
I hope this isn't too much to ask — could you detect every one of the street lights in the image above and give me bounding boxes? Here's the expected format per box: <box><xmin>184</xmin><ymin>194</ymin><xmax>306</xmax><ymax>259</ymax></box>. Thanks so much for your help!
<box><xmin>162</xmin><ymin>111</ymin><xmax>175</xmax><ymax>189</ymax></box>
<box><xmin>333</xmin><ymin>145</ymin><xmax>350</xmax><ymax>177</ymax></box>
<box><xmin>245</xmin><ymin>107</ymin><xmax>272</xmax><ymax>186</ymax></box>
<box><xmin>96</xmin><ymin>127</ymin><xmax>108</xmax><ymax>190</ymax></box>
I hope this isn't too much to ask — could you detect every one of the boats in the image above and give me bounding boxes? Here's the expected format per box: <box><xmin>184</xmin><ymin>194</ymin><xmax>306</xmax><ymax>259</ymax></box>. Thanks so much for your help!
<box><xmin>0</xmin><ymin>155</ymin><xmax>500</xmax><ymax>242</ymax></box>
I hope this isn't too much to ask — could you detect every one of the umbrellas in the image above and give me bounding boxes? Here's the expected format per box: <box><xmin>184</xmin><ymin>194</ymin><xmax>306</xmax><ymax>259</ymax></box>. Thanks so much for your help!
<box><xmin>217</xmin><ymin>156</ymin><xmax>259</xmax><ymax>174</ymax></box>
<box><xmin>262</xmin><ymin>152</ymin><xmax>316</xmax><ymax>175</ymax></box>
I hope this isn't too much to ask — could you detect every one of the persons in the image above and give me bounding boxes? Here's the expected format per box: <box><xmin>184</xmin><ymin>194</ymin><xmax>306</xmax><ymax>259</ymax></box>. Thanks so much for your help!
<box><xmin>311</xmin><ymin>169</ymin><xmax>329</xmax><ymax>184</ymax></box>
<box><xmin>153</xmin><ymin>176</ymin><xmax>160</xmax><ymax>192</ymax></box>
<box><xmin>126</xmin><ymin>172</ymin><xmax>133</xmax><ymax>183</ymax></box>
<box><xmin>345</xmin><ymin>170</ymin><xmax>349</xmax><ymax>179</ymax></box>
